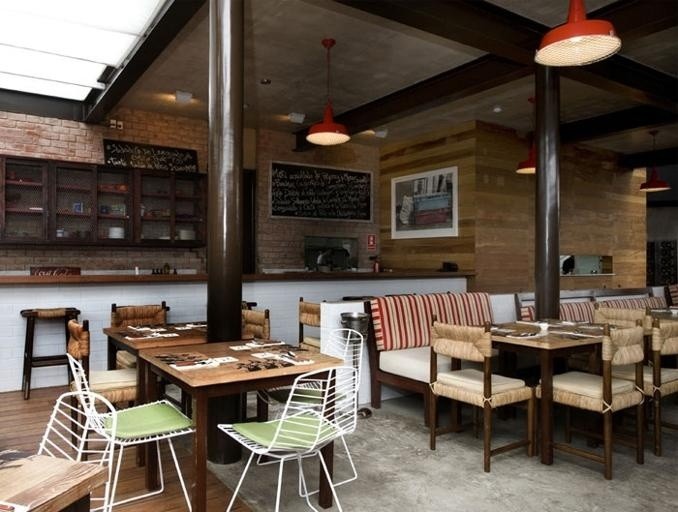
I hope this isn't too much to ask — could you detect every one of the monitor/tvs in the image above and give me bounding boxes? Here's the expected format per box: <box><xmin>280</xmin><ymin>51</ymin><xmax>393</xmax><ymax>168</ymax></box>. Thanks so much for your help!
<box><xmin>305</xmin><ymin>236</ymin><xmax>359</xmax><ymax>268</ymax></box>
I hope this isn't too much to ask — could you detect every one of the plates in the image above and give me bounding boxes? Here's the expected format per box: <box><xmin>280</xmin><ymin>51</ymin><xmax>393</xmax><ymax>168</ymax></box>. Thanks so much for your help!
<box><xmin>107</xmin><ymin>225</ymin><xmax>126</xmax><ymax>240</ymax></box>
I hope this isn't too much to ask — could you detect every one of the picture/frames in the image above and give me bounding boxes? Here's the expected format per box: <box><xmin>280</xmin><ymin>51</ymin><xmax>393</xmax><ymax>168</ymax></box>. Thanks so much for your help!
<box><xmin>390</xmin><ymin>166</ymin><xmax>459</xmax><ymax>239</ymax></box>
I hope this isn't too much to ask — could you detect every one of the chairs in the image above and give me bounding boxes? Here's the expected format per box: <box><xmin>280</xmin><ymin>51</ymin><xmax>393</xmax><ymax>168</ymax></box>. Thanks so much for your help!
<box><xmin>257</xmin><ymin>329</ymin><xmax>365</xmax><ymax>497</ymax></box>
<box><xmin>66</xmin><ymin>353</ymin><xmax>195</xmax><ymax>511</ymax></box>
<box><xmin>298</xmin><ymin>297</ymin><xmax>327</xmax><ymax>355</ymax></box>
<box><xmin>589</xmin><ymin>312</ymin><xmax>678</xmax><ymax>457</ymax></box>
<box><xmin>38</xmin><ymin>391</ymin><xmax>117</xmax><ymax>512</ymax></box>
<box><xmin>65</xmin><ymin>311</ymin><xmax>141</xmax><ymax>461</ymax></box>
<box><xmin>536</xmin><ymin>319</ymin><xmax>645</xmax><ymax>479</ymax></box>
<box><xmin>230</xmin><ymin>305</ymin><xmax>270</xmax><ymax>422</ymax></box>
<box><xmin>427</xmin><ymin>313</ymin><xmax>536</xmax><ymax>474</ymax></box>
<box><xmin>215</xmin><ymin>368</ymin><xmax>361</xmax><ymax>512</ymax></box>
<box><xmin>577</xmin><ymin>304</ymin><xmax>652</xmax><ymax>376</ymax></box>
<box><xmin>111</xmin><ymin>300</ymin><xmax>170</xmax><ymax>370</ymax></box>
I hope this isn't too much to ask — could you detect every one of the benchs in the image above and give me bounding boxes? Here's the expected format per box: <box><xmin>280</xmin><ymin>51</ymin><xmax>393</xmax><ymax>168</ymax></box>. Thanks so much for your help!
<box><xmin>664</xmin><ymin>282</ymin><xmax>678</xmax><ymax>308</ymax></box>
<box><xmin>514</xmin><ymin>287</ymin><xmax>667</xmax><ymax>371</ymax></box>
<box><xmin>364</xmin><ymin>292</ymin><xmax>500</xmax><ymax>429</ymax></box>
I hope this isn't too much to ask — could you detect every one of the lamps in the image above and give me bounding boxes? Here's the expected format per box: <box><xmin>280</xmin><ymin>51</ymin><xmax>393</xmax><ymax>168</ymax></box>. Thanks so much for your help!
<box><xmin>640</xmin><ymin>130</ymin><xmax>670</xmax><ymax>192</ymax></box>
<box><xmin>516</xmin><ymin>97</ymin><xmax>536</xmax><ymax>176</ymax></box>
<box><xmin>305</xmin><ymin>37</ymin><xmax>352</xmax><ymax>148</ymax></box>
<box><xmin>534</xmin><ymin>0</ymin><xmax>623</xmax><ymax>67</ymax></box>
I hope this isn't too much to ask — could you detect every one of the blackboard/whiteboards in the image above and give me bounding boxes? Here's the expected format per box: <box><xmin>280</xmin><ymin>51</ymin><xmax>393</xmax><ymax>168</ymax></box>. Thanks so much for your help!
<box><xmin>267</xmin><ymin>159</ymin><xmax>374</xmax><ymax>225</ymax></box>
<box><xmin>103</xmin><ymin>138</ymin><xmax>198</xmax><ymax>174</ymax></box>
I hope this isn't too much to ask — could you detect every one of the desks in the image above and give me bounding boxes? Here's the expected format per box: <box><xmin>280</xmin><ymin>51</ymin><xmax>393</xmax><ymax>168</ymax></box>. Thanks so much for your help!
<box><xmin>103</xmin><ymin>319</ymin><xmax>207</xmax><ymax>468</ymax></box>
<box><xmin>0</xmin><ymin>446</ymin><xmax>110</xmax><ymax>512</ymax></box>
<box><xmin>448</xmin><ymin>317</ymin><xmax>652</xmax><ymax>465</ymax></box>
<box><xmin>140</xmin><ymin>338</ymin><xmax>346</xmax><ymax>510</ymax></box>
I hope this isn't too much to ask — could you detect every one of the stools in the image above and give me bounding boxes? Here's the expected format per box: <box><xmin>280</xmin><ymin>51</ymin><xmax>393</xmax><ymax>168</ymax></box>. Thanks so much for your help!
<box><xmin>240</xmin><ymin>299</ymin><xmax>257</xmax><ymax>310</ymax></box>
<box><xmin>19</xmin><ymin>306</ymin><xmax>82</xmax><ymax>400</ymax></box>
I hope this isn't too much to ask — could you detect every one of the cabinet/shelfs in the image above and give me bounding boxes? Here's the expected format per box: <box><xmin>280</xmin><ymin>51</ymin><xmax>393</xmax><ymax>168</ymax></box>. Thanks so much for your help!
<box><xmin>0</xmin><ymin>154</ymin><xmax>207</xmax><ymax>252</ymax></box>
